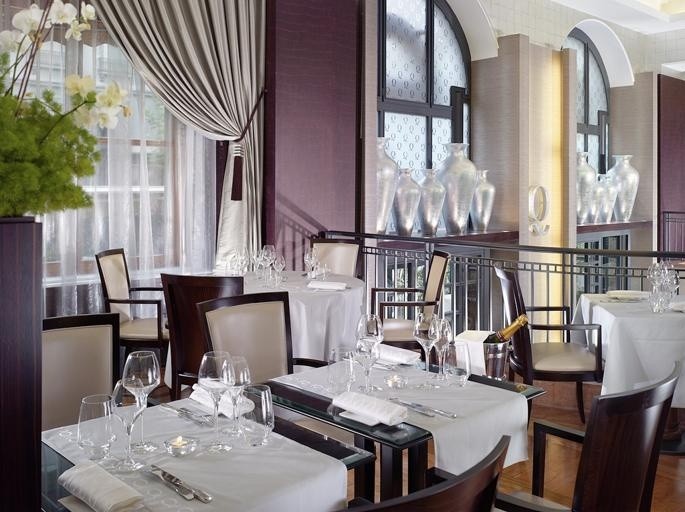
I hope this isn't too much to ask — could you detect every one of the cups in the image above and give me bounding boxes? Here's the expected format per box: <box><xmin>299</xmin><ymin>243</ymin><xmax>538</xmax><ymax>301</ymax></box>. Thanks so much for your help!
<box><xmin>304</xmin><ymin>253</ymin><xmax>315</xmax><ymax>281</ymax></box>
<box><xmin>661</xmin><ymin>261</ymin><xmax>676</xmax><ymax>286</ymax></box>
<box><xmin>308</xmin><ymin>246</ymin><xmax>319</xmax><ymax>276</ymax></box>
<box><xmin>248</xmin><ymin>251</ymin><xmax>259</xmax><ymax>280</ymax></box>
<box><xmin>259</xmin><ymin>251</ymin><xmax>272</xmax><ymax>284</ymax></box>
<box><xmin>223</xmin><ymin>356</ymin><xmax>251</xmax><ymax>436</ymax></box>
<box><xmin>76</xmin><ymin>394</ymin><xmax>114</xmax><ymax>463</ymax></box>
<box><xmin>356</xmin><ymin>314</ymin><xmax>384</xmax><ymax>391</ymax></box>
<box><xmin>240</xmin><ymin>385</ymin><xmax>274</xmax><ymax>446</ymax></box>
<box><xmin>412</xmin><ymin>313</ymin><xmax>440</xmax><ymax>389</ymax></box>
<box><xmin>271</xmin><ymin>255</ymin><xmax>286</xmax><ymax>288</ymax></box>
<box><xmin>262</xmin><ymin>244</ymin><xmax>276</xmax><ymax>280</ymax></box>
<box><xmin>354</xmin><ymin>339</ymin><xmax>380</xmax><ymax>396</ymax></box>
<box><xmin>320</xmin><ymin>263</ymin><xmax>330</xmax><ymax>281</ymax></box>
<box><xmin>662</xmin><ymin>270</ymin><xmax>680</xmax><ymax>296</ymax></box>
<box><xmin>197</xmin><ymin>351</ymin><xmax>234</xmax><ymax>454</ymax></box>
<box><xmin>254</xmin><ymin>250</ymin><xmax>262</xmax><ymax>284</ymax></box>
<box><xmin>442</xmin><ymin>342</ymin><xmax>471</xmax><ymax>389</ymax></box>
<box><xmin>121</xmin><ymin>351</ymin><xmax>160</xmax><ymax>454</ymax></box>
<box><xmin>646</xmin><ymin>265</ymin><xmax>661</xmax><ymax>293</ymax></box>
<box><xmin>648</xmin><ymin>282</ymin><xmax>666</xmax><ymax>314</ymax></box>
<box><xmin>427</xmin><ymin>317</ymin><xmax>452</xmax><ymax>381</ymax></box>
<box><xmin>328</xmin><ymin>348</ymin><xmax>353</xmax><ymax>392</ymax></box>
<box><xmin>109</xmin><ymin>377</ymin><xmax>147</xmax><ymax>473</ymax></box>
<box><xmin>483</xmin><ymin>341</ymin><xmax>508</xmax><ymax>381</ymax></box>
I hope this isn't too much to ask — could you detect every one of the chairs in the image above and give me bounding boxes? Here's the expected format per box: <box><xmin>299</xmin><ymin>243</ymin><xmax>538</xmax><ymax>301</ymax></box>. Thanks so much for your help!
<box><xmin>161</xmin><ymin>273</ymin><xmax>244</xmax><ymax>401</ymax></box>
<box><xmin>196</xmin><ymin>292</ymin><xmax>333</xmax><ymax>384</ymax></box>
<box><xmin>42</xmin><ymin>313</ymin><xmax>120</xmax><ymax>431</ymax></box>
<box><xmin>494</xmin><ymin>267</ymin><xmax>606</xmax><ymax>430</ymax></box>
<box><xmin>310</xmin><ymin>238</ymin><xmax>363</xmax><ymax>279</ymax></box>
<box><xmin>371</xmin><ymin>250</ymin><xmax>450</xmax><ymax>362</ymax></box>
<box><xmin>494</xmin><ymin>362</ymin><xmax>681</xmax><ymax>512</ymax></box>
<box><xmin>95</xmin><ymin>248</ymin><xmax>169</xmax><ymax>367</ymax></box>
<box><xmin>344</xmin><ymin>435</ymin><xmax>511</xmax><ymax>512</ymax></box>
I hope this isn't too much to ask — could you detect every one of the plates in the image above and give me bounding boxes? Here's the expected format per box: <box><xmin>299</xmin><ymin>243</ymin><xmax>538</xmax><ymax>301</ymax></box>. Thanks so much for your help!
<box><xmin>670</xmin><ymin>302</ymin><xmax>685</xmax><ymax>312</ymax></box>
<box><xmin>607</xmin><ymin>290</ymin><xmax>649</xmax><ymax>299</ymax></box>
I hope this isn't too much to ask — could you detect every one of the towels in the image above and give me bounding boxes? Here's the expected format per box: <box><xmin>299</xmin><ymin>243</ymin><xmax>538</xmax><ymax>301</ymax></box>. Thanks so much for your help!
<box><xmin>58</xmin><ymin>459</ymin><xmax>144</xmax><ymax>512</ymax></box>
<box><xmin>306</xmin><ymin>279</ymin><xmax>348</xmax><ymax>291</ymax></box>
<box><xmin>191</xmin><ymin>377</ymin><xmax>255</xmax><ymax>420</ymax></box>
<box><xmin>333</xmin><ymin>391</ymin><xmax>407</xmax><ymax>426</ymax></box>
<box><xmin>372</xmin><ymin>341</ymin><xmax>421</xmax><ymax>366</ymax></box>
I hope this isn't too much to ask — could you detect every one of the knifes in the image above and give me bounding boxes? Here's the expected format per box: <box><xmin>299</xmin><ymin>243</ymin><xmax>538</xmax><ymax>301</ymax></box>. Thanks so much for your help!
<box><xmin>396</xmin><ymin>397</ymin><xmax>457</xmax><ymax>418</ymax></box>
<box><xmin>160</xmin><ymin>402</ymin><xmax>204</xmax><ymax>426</ymax></box>
<box><xmin>390</xmin><ymin>398</ymin><xmax>434</xmax><ymax>417</ymax></box>
<box><xmin>150</xmin><ymin>463</ymin><xmax>213</xmax><ymax>504</ymax></box>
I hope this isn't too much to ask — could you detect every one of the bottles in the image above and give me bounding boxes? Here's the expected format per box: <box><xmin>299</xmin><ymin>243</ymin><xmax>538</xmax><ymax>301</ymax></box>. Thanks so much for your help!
<box><xmin>483</xmin><ymin>316</ymin><xmax>528</xmax><ymax>342</ymax></box>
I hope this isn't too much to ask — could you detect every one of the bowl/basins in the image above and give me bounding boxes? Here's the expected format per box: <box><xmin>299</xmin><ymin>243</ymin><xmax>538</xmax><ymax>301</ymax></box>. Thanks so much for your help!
<box><xmin>384</xmin><ymin>375</ymin><xmax>408</xmax><ymax>389</ymax></box>
<box><xmin>163</xmin><ymin>435</ymin><xmax>197</xmax><ymax>456</ymax></box>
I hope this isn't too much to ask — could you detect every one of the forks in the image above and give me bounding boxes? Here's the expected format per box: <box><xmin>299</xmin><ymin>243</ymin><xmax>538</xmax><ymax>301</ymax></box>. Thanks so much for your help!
<box><xmin>139</xmin><ymin>465</ymin><xmax>195</xmax><ymax>502</ymax></box>
<box><xmin>180</xmin><ymin>406</ymin><xmax>215</xmax><ymax>425</ymax></box>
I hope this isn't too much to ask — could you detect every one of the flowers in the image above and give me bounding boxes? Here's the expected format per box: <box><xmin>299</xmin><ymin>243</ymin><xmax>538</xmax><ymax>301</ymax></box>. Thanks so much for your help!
<box><xmin>0</xmin><ymin>0</ymin><xmax>133</xmax><ymax>217</ymax></box>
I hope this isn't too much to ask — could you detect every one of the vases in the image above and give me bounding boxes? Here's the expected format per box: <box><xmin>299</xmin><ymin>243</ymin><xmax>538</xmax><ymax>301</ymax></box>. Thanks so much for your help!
<box><xmin>607</xmin><ymin>155</ymin><xmax>639</xmax><ymax>222</ymax></box>
<box><xmin>470</xmin><ymin>170</ymin><xmax>496</xmax><ymax>231</ymax></box>
<box><xmin>0</xmin><ymin>216</ymin><xmax>34</xmax><ymax>223</ymax></box>
<box><xmin>439</xmin><ymin>143</ymin><xmax>478</xmax><ymax>235</ymax></box>
<box><xmin>393</xmin><ymin>169</ymin><xmax>421</xmax><ymax>236</ymax></box>
<box><xmin>600</xmin><ymin>174</ymin><xmax>617</xmax><ymax>223</ymax></box>
<box><xmin>588</xmin><ymin>174</ymin><xmax>607</xmax><ymax>223</ymax></box>
<box><xmin>577</xmin><ymin>152</ymin><xmax>597</xmax><ymax>224</ymax></box>
<box><xmin>417</xmin><ymin>169</ymin><xmax>446</xmax><ymax>236</ymax></box>
<box><xmin>376</xmin><ymin>137</ymin><xmax>399</xmax><ymax>234</ymax></box>
